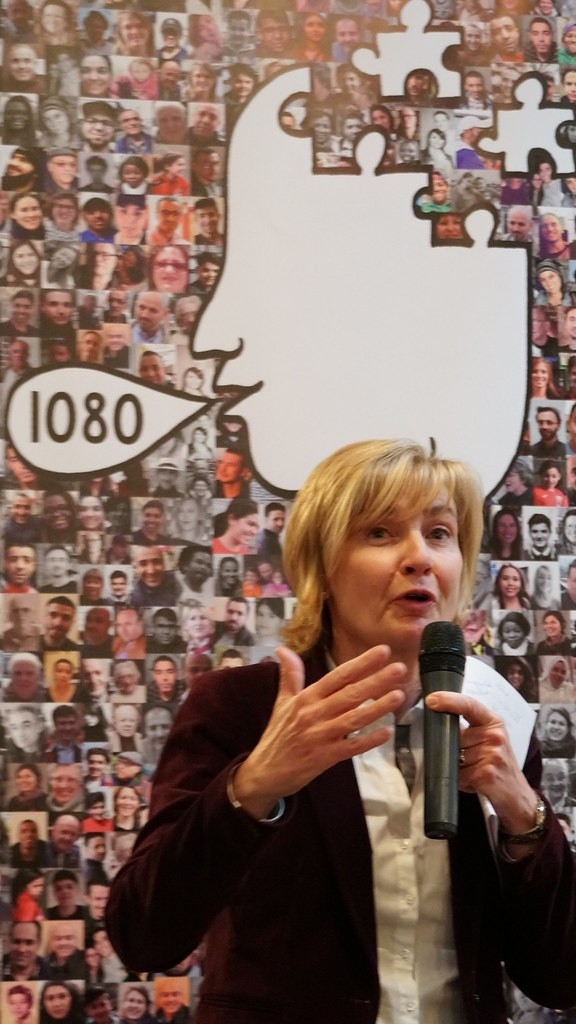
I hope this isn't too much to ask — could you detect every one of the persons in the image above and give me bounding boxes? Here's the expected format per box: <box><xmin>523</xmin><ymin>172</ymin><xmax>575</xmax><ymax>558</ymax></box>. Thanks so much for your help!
<box><xmin>100</xmin><ymin>439</ymin><xmax>575</xmax><ymax>1024</ymax></box>
<box><xmin>79</xmin><ymin>156</ymin><xmax>112</xmax><ymax>193</ymax></box>
<box><xmin>150</xmin><ymin>152</ymin><xmax>190</xmax><ymax>196</ymax></box>
<box><xmin>192</xmin><ymin>149</ymin><xmax>224</xmax><ymax>197</ymax></box>
<box><xmin>156</xmin><ymin>19</ymin><xmax>187</xmax><ymax>59</ymax></box>
<box><xmin>42</xmin><ymin>192</ymin><xmax>79</xmax><ymax>240</ymax></box>
<box><xmin>116</xmin><ymin>110</ymin><xmax>151</xmax><ymax>154</ymax></box>
<box><xmin>189</xmin><ymin>14</ymin><xmax>221</xmax><ymax>60</ymax></box>
<box><xmin>159</xmin><ymin>61</ymin><xmax>182</xmax><ymax>100</ymax></box>
<box><xmin>149</xmin><ymin>244</ymin><xmax>188</xmax><ymax>293</ymax></box>
<box><xmin>78</xmin><ymin>55</ymin><xmax>116</xmax><ymax>99</ymax></box>
<box><xmin>115</xmin><ymin>155</ymin><xmax>150</xmax><ymax>194</ymax></box>
<box><xmin>80</xmin><ymin>198</ymin><xmax>115</xmax><ymax>242</ymax></box>
<box><xmin>194</xmin><ymin>198</ymin><xmax>225</xmax><ymax>245</ymax></box>
<box><xmin>131</xmin><ymin>291</ymin><xmax>171</xmax><ymax>344</ymax></box>
<box><xmin>0</xmin><ymin>1</ymin><xmax>576</xmax><ymax>1024</ymax></box>
<box><xmin>39</xmin><ymin>96</ymin><xmax>78</xmax><ymax>148</ymax></box>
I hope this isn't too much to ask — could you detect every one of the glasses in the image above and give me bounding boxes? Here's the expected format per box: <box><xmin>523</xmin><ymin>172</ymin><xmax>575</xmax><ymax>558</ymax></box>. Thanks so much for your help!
<box><xmin>536</xmin><ymin>419</ymin><xmax>559</xmax><ymax>425</ymax></box>
<box><xmin>82</xmin><ymin>116</ymin><xmax>117</xmax><ymax>127</ymax></box>
<box><xmin>155</xmin><ymin>261</ymin><xmax>187</xmax><ymax>268</ymax></box>
<box><xmin>90</xmin><ymin>251</ymin><xmax>120</xmax><ymax>258</ymax></box>
<box><xmin>51</xmin><ymin>159</ymin><xmax>79</xmax><ymax>170</ymax></box>
<box><xmin>11</xmin><ymin>938</ymin><xmax>33</xmax><ymax>945</ymax></box>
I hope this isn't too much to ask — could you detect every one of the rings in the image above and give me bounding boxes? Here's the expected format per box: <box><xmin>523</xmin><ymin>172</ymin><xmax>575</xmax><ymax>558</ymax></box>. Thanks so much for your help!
<box><xmin>459</xmin><ymin>749</ymin><xmax>465</xmax><ymax>766</ymax></box>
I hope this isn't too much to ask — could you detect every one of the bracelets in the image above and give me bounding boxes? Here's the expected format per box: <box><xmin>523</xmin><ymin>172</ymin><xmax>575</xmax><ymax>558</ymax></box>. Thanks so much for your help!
<box><xmin>227</xmin><ymin>761</ymin><xmax>286</xmax><ymax>823</ymax></box>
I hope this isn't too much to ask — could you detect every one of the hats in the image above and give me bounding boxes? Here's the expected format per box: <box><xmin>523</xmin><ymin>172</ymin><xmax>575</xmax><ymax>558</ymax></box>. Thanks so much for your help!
<box><xmin>82</xmin><ymin>100</ymin><xmax>123</xmax><ymax>117</ymax></box>
<box><xmin>457</xmin><ymin>116</ymin><xmax>494</xmax><ymax>135</ymax></box>
<box><xmin>536</xmin><ymin>258</ymin><xmax>569</xmax><ymax>276</ymax></box>
<box><xmin>39</xmin><ymin>95</ymin><xmax>74</xmax><ymax>115</ymax></box>
<box><xmin>117</xmin><ymin>194</ymin><xmax>146</xmax><ymax>208</ymax></box>
<box><xmin>13</xmin><ymin>145</ymin><xmax>47</xmax><ymax>167</ymax></box>
<box><xmin>160</xmin><ymin>18</ymin><xmax>183</xmax><ymax>35</ymax></box>
<box><xmin>561</xmin><ymin>21</ymin><xmax>576</xmax><ymax>36</ymax></box>
<box><xmin>116</xmin><ymin>750</ymin><xmax>142</xmax><ymax>765</ymax></box>
<box><xmin>46</xmin><ymin>147</ymin><xmax>78</xmax><ymax>157</ymax></box>
<box><xmin>150</xmin><ymin>457</ymin><xmax>181</xmax><ymax>471</ymax></box>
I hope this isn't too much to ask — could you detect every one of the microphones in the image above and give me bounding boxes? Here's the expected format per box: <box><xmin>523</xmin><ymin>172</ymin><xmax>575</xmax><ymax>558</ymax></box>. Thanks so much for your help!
<box><xmin>417</xmin><ymin>621</ymin><xmax>467</xmax><ymax>839</ymax></box>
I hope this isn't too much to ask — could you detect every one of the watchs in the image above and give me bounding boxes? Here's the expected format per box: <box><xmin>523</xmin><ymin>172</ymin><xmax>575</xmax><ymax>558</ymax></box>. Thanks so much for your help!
<box><xmin>499</xmin><ymin>796</ymin><xmax>549</xmax><ymax>843</ymax></box>
